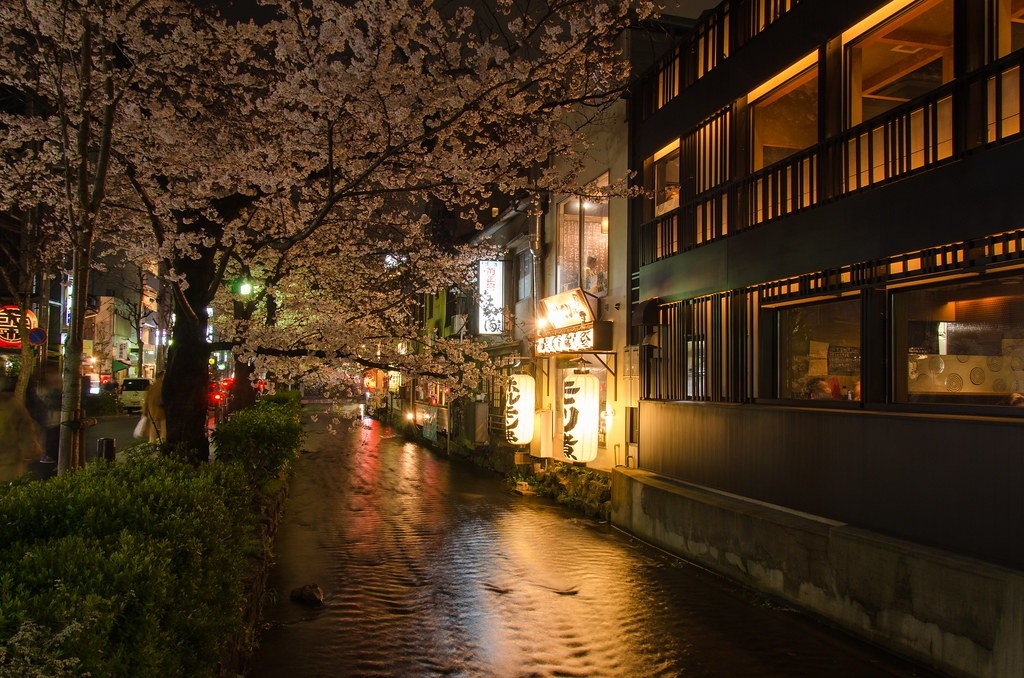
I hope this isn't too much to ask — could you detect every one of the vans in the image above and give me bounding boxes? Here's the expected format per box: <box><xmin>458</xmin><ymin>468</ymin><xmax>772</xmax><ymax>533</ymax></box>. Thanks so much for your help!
<box><xmin>116</xmin><ymin>378</ymin><xmax>154</xmax><ymax>414</ymax></box>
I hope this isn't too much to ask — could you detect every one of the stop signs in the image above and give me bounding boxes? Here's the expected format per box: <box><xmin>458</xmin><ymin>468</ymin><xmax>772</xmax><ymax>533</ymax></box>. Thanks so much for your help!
<box><xmin>28</xmin><ymin>328</ymin><xmax>48</xmax><ymax>345</ymax></box>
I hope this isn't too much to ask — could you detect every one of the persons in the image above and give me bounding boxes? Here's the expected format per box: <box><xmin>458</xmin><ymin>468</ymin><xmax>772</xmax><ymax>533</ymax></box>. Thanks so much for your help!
<box><xmin>801</xmin><ymin>375</ymin><xmax>834</xmax><ymax>403</ymax></box>
<box><xmin>141</xmin><ymin>369</ymin><xmax>169</xmax><ymax>446</ymax></box>
<box><xmin>850</xmin><ymin>375</ymin><xmax>860</xmax><ymax>401</ymax></box>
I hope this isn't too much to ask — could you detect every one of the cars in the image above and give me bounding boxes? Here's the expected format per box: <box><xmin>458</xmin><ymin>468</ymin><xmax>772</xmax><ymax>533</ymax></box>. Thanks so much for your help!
<box><xmin>206</xmin><ymin>378</ymin><xmax>234</xmax><ymax>410</ymax></box>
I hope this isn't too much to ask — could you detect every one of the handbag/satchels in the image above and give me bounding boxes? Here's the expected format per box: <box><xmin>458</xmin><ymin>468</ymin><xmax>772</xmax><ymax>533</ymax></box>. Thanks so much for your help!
<box><xmin>133</xmin><ymin>417</ymin><xmax>147</xmax><ymax>438</ymax></box>
